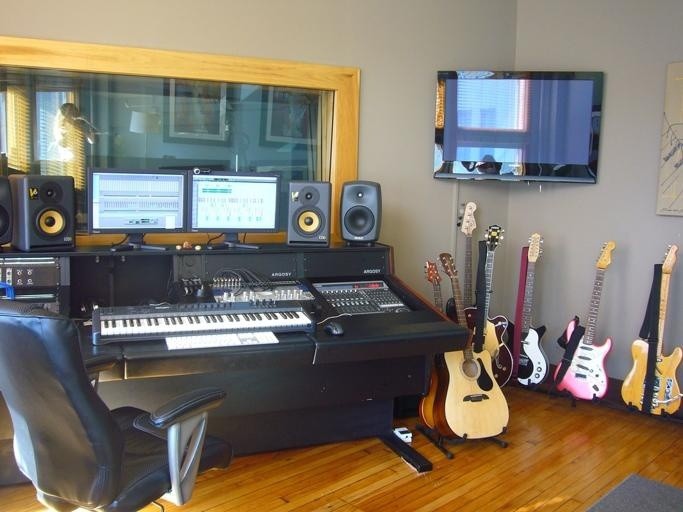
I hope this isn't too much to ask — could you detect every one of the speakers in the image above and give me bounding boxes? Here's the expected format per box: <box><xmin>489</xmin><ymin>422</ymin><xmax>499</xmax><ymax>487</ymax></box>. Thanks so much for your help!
<box><xmin>287</xmin><ymin>181</ymin><xmax>332</xmax><ymax>247</ymax></box>
<box><xmin>339</xmin><ymin>181</ymin><xmax>382</xmax><ymax>248</ymax></box>
<box><xmin>0</xmin><ymin>176</ymin><xmax>13</xmax><ymax>252</ymax></box>
<box><xmin>8</xmin><ymin>174</ymin><xmax>75</xmax><ymax>252</ymax></box>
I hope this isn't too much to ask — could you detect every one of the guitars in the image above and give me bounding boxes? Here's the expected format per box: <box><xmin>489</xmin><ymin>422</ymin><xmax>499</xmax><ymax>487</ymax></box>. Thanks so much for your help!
<box><xmin>620</xmin><ymin>245</ymin><xmax>682</xmax><ymax>417</ymax></box>
<box><xmin>420</xmin><ymin>201</ymin><xmax>549</xmax><ymax>438</ymax></box>
<box><xmin>553</xmin><ymin>239</ymin><xmax>616</xmax><ymax>402</ymax></box>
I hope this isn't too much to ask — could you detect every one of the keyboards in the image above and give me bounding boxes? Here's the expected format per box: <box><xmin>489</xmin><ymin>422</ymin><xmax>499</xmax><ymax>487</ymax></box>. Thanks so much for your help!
<box><xmin>165</xmin><ymin>331</ymin><xmax>280</xmax><ymax>351</ymax></box>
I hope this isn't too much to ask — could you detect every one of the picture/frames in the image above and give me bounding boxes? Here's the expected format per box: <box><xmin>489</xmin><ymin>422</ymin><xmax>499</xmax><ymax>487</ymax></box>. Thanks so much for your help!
<box><xmin>162</xmin><ymin>77</ymin><xmax>235</xmax><ymax>146</ymax></box>
<box><xmin>248</xmin><ymin>159</ymin><xmax>310</xmax><ymax>181</ymax></box>
<box><xmin>259</xmin><ymin>84</ymin><xmax>318</xmax><ymax>148</ymax></box>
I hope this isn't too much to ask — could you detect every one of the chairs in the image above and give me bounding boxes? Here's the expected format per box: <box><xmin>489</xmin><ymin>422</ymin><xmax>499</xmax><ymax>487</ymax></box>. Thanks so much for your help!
<box><xmin>0</xmin><ymin>284</ymin><xmax>236</xmax><ymax>512</ymax></box>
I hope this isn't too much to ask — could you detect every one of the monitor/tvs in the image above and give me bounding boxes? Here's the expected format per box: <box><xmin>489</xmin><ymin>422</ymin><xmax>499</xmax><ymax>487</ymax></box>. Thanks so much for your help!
<box><xmin>188</xmin><ymin>169</ymin><xmax>280</xmax><ymax>249</ymax></box>
<box><xmin>87</xmin><ymin>167</ymin><xmax>188</xmax><ymax>251</ymax></box>
<box><xmin>433</xmin><ymin>70</ymin><xmax>604</xmax><ymax>184</ymax></box>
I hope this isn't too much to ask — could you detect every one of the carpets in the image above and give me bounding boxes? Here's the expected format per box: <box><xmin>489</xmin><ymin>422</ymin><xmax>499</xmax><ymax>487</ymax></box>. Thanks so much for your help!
<box><xmin>589</xmin><ymin>471</ymin><xmax>683</xmax><ymax>511</ymax></box>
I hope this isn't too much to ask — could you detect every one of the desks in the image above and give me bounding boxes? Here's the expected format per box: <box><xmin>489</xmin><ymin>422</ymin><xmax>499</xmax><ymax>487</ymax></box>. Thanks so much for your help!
<box><xmin>0</xmin><ymin>239</ymin><xmax>476</xmax><ymax>488</ymax></box>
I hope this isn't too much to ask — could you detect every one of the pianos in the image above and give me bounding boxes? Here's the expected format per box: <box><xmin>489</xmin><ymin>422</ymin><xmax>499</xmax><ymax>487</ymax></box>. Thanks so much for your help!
<box><xmin>91</xmin><ymin>298</ymin><xmax>315</xmax><ymax>347</ymax></box>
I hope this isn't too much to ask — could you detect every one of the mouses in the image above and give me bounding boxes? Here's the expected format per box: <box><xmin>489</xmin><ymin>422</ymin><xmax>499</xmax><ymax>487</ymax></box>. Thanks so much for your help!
<box><xmin>323</xmin><ymin>322</ymin><xmax>344</xmax><ymax>337</ymax></box>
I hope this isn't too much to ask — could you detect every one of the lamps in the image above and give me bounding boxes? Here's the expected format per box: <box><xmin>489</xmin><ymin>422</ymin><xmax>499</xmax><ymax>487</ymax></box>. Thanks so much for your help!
<box><xmin>129</xmin><ymin>110</ymin><xmax>162</xmax><ymax>157</ymax></box>
